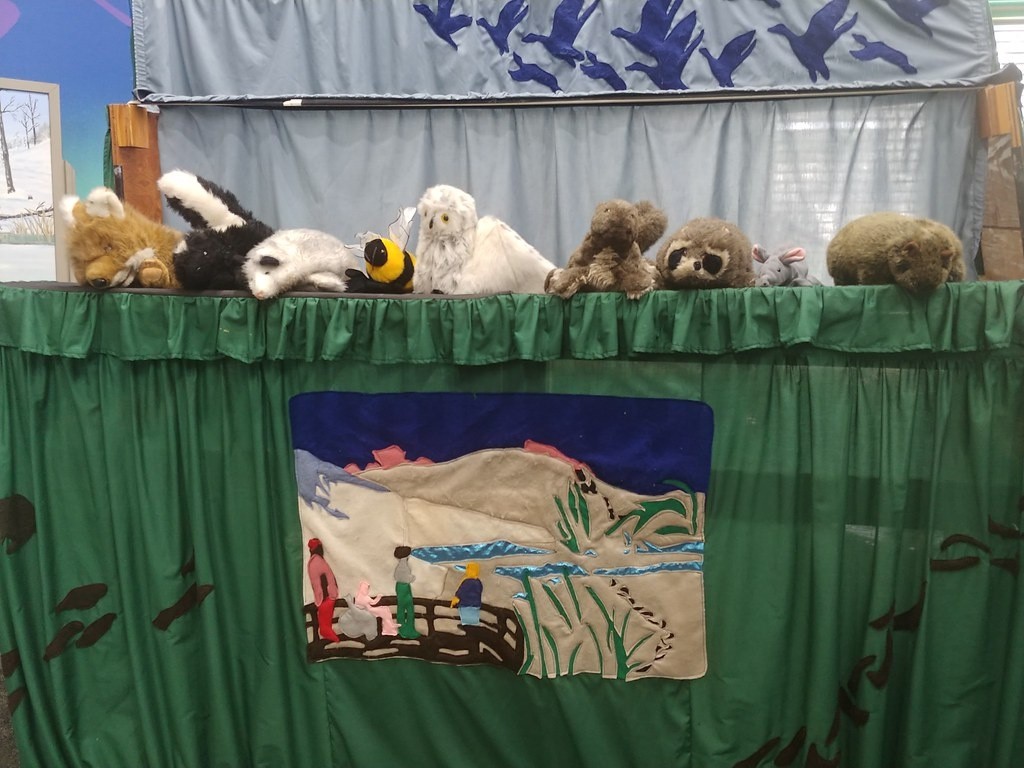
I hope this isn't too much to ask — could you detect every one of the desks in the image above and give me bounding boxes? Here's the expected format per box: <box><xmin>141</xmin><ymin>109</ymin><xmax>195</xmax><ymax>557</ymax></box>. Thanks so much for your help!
<box><xmin>0</xmin><ymin>279</ymin><xmax>1024</xmax><ymax>768</ymax></box>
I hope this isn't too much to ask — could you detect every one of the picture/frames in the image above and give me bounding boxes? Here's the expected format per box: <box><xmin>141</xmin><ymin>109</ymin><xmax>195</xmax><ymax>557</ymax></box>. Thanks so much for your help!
<box><xmin>0</xmin><ymin>77</ymin><xmax>70</xmax><ymax>283</ymax></box>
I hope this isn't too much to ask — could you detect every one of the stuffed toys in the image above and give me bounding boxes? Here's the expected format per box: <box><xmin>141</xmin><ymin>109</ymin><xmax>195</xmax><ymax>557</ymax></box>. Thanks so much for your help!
<box><xmin>752</xmin><ymin>243</ymin><xmax>824</xmax><ymax>287</ymax></box>
<box><xmin>60</xmin><ymin>170</ymin><xmax>966</xmax><ymax>298</ymax></box>
